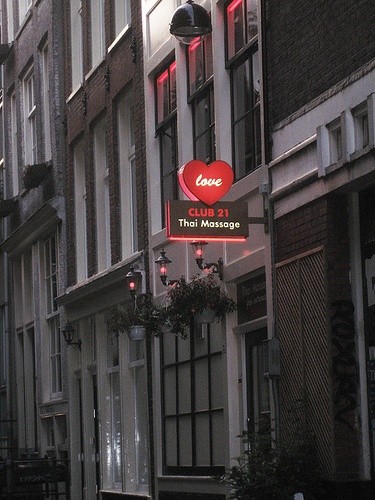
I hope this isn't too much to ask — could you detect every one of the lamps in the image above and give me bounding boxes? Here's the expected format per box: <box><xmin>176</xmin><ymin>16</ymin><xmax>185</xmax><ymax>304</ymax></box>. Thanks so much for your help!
<box><xmin>124</xmin><ymin>267</ymin><xmax>150</xmax><ymax>306</ymax></box>
<box><xmin>61</xmin><ymin>320</ymin><xmax>81</xmax><ymax>351</ymax></box>
<box><xmin>190</xmin><ymin>239</ymin><xmax>223</xmax><ymax>280</ymax></box>
<box><xmin>155</xmin><ymin>249</ymin><xmax>185</xmax><ymax>286</ymax></box>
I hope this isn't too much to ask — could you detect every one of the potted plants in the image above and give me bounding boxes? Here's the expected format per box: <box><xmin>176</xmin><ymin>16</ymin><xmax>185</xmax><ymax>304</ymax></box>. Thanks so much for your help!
<box><xmin>164</xmin><ymin>271</ymin><xmax>238</xmax><ymax>341</ymax></box>
<box><xmin>170</xmin><ymin>1</ymin><xmax>212</xmax><ymax>45</ymax></box>
<box><xmin>106</xmin><ymin>300</ymin><xmax>164</xmax><ymax>341</ymax></box>
